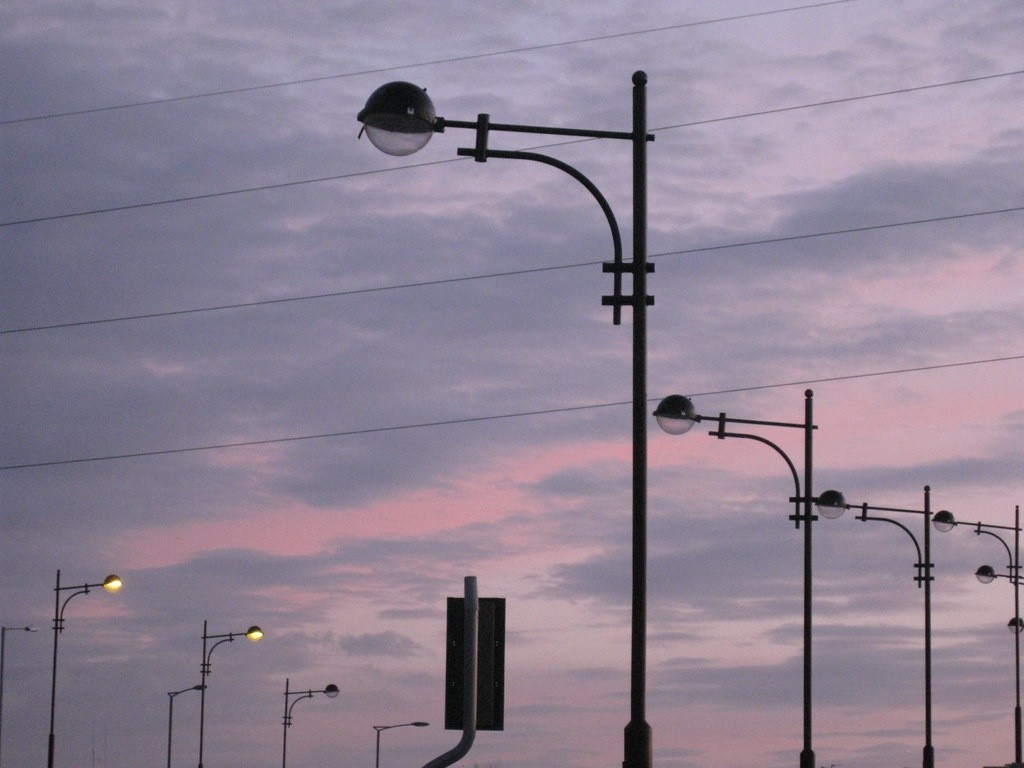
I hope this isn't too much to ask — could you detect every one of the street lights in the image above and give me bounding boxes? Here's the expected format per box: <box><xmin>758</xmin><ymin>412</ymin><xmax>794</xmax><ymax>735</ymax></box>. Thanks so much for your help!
<box><xmin>937</xmin><ymin>513</ymin><xmax>1021</xmax><ymax>768</ymax></box>
<box><xmin>0</xmin><ymin>626</ymin><xmax>38</xmax><ymax>730</ymax></box>
<box><xmin>282</xmin><ymin>684</ymin><xmax>339</xmax><ymax>768</ymax></box>
<box><xmin>654</xmin><ymin>395</ymin><xmax>814</xmax><ymax>767</ymax></box>
<box><xmin>197</xmin><ymin>626</ymin><xmax>264</xmax><ymax>768</ymax></box>
<box><xmin>355</xmin><ymin>81</ymin><xmax>653</xmax><ymax>768</ymax></box>
<box><xmin>47</xmin><ymin>573</ymin><xmax>123</xmax><ymax>768</ymax></box>
<box><xmin>376</xmin><ymin>722</ymin><xmax>428</xmax><ymax>768</ymax></box>
<box><xmin>817</xmin><ymin>489</ymin><xmax>935</xmax><ymax>768</ymax></box>
<box><xmin>167</xmin><ymin>684</ymin><xmax>207</xmax><ymax>767</ymax></box>
